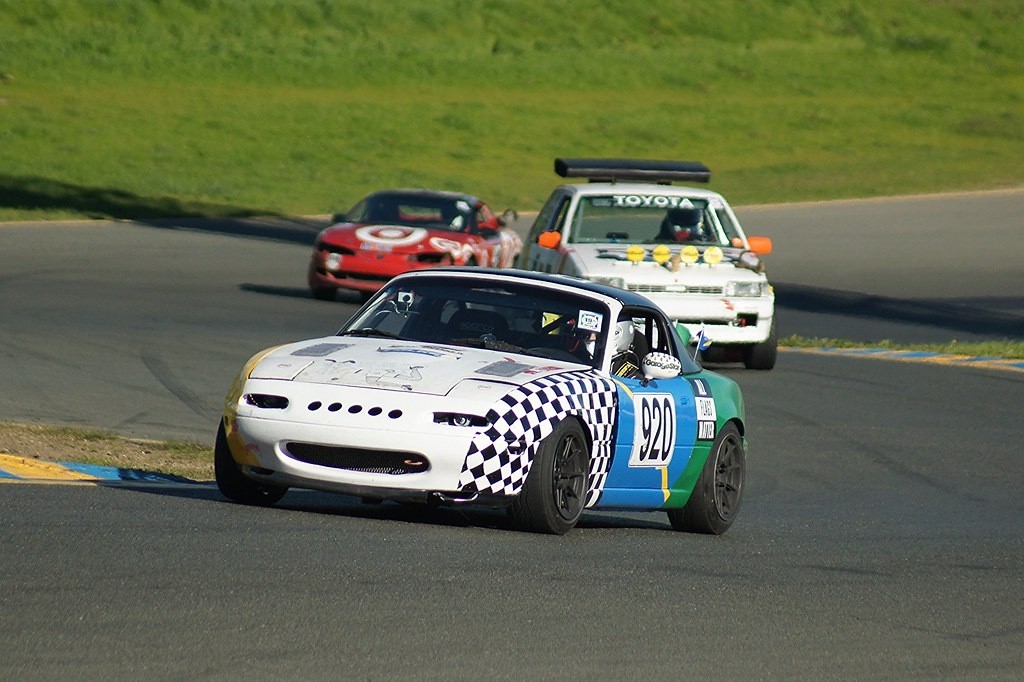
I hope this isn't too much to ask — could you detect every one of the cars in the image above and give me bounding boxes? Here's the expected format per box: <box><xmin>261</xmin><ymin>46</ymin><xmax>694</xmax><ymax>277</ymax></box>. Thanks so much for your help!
<box><xmin>212</xmin><ymin>264</ymin><xmax>749</xmax><ymax>538</ymax></box>
<box><xmin>514</xmin><ymin>155</ymin><xmax>781</xmax><ymax>372</ymax></box>
<box><xmin>307</xmin><ymin>188</ymin><xmax>526</xmax><ymax>306</ymax></box>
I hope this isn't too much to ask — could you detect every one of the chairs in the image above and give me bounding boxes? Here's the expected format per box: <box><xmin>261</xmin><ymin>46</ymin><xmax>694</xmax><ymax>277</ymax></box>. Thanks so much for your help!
<box><xmin>441</xmin><ymin>306</ymin><xmax>507</xmax><ymax>346</ymax></box>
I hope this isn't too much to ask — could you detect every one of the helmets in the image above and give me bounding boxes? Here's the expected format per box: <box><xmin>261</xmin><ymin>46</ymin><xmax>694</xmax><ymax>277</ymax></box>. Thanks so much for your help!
<box><xmin>667</xmin><ymin>209</ymin><xmax>703</xmax><ymax>238</ymax></box>
<box><xmin>583</xmin><ymin>320</ymin><xmax>635</xmax><ymax>359</ymax></box>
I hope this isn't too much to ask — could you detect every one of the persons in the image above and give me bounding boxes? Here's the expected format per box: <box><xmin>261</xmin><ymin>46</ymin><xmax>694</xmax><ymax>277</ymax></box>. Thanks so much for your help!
<box><xmin>665</xmin><ymin>208</ymin><xmax>702</xmax><ymax>241</ymax></box>
<box><xmin>583</xmin><ymin>317</ymin><xmax>645</xmax><ymax>380</ymax></box>
